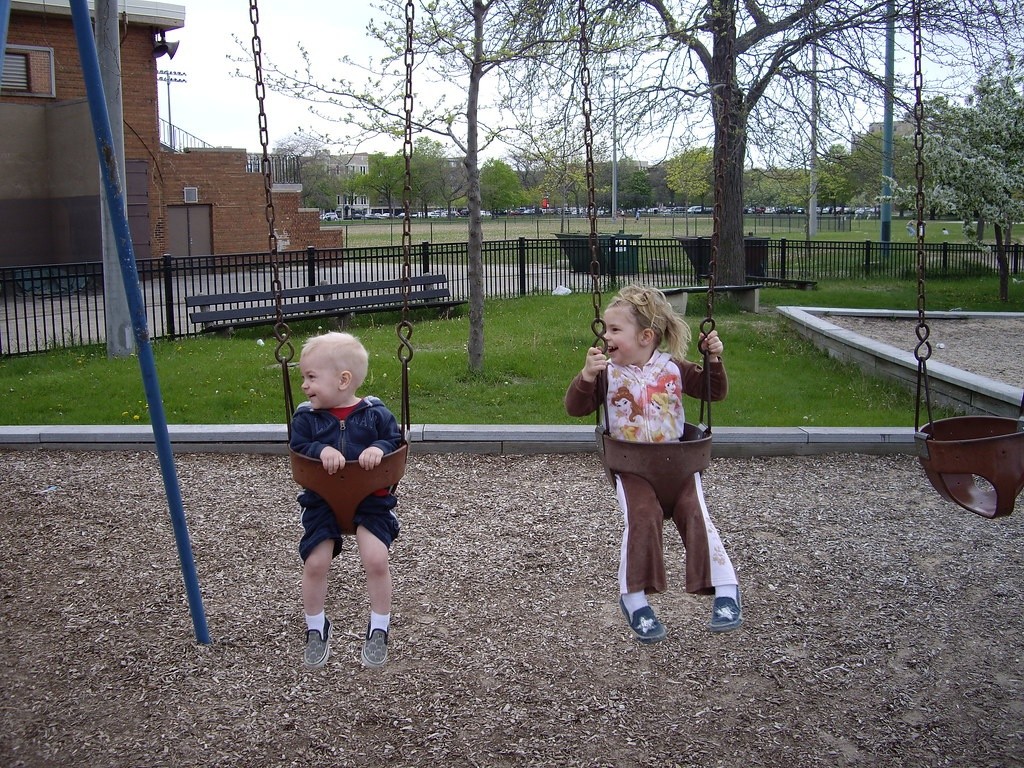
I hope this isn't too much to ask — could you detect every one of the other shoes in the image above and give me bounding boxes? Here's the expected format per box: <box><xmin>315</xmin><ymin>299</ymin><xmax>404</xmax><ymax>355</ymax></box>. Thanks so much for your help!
<box><xmin>304</xmin><ymin>618</ymin><xmax>331</xmax><ymax>670</ymax></box>
<box><xmin>361</xmin><ymin>622</ymin><xmax>390</xmax><ymax>669</ymax></box>
<box><xmin>619</xmin><ymin>595</ymin><xmax>665</xmax><ymax>643</ymax></box>
<box><xmin>710</xmin><ymin>586</ymin><xmax>741</xmax><ymax>632</ymax></box>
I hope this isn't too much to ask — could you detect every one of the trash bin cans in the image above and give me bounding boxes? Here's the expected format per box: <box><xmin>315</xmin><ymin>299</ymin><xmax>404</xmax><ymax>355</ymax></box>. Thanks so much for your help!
<box><xmin>654</xmin><ymin>209</ymin><xmax>658</xmax><ymax>214</ymax></box>
<box><xmin>555</xmin><ymin>230</ymin><xmax>642</xmax><ymax>274</ymax></box>
<box><xmin>677</xmin><ymin>232</ymin><xmax>770</xmax><ymax>278</ymax></box>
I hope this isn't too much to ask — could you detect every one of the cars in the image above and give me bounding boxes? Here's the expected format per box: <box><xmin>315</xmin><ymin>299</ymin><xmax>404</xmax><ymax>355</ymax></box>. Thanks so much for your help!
<box><xmin>359</xmin><ymin>205</ymin><xmax>880</xmax><ymax>222</ymax></box>
<box><xmin>320</xmin><ymin>211</ymin><xmax>339</xmax><ymax>222</ymax></box>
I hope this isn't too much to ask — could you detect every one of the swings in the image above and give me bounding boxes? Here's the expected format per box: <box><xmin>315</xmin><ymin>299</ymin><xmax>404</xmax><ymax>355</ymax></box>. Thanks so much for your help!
<box><xmin>912</xmin><ymin>0</ymin><xmax>1024</xmax><ymax>520</ymax></box>
<box><xmin>577</xmin><ymin>0</ymin><xmax>739</xmax><ymax>521</ymax></box>
<box><xmin>249</xmin><ymin>0</ymin><xmax>414</xmax><ymax>536</ymax></box>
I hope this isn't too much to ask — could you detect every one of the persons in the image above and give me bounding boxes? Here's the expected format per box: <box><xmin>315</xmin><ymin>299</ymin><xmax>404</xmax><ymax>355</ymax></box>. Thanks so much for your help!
<box><xmin>906</xmin><ymin>217</ymin><xmax>917</xmax><ymax>237</ymax></box>
<box><xmin>290</xmin><ymin>333</ymin><xmax>405</xmax><ymax>671</ymax></box>
<box><xmin>565</xmin><ymin>285</ymin><xmax>742</xmax><ymax>643</ymax></box>
<box><xmin>636</xmin><ymin>211</ymin><xmax>639</xmax><ymax>220</ymax></box>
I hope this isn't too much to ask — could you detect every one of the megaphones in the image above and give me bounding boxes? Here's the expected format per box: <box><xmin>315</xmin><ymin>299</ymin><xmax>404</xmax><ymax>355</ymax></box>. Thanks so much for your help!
<box><xmin>152</xmin><ymin>40</ymin><xmax>180</xmax><ymax>60</ymax></box>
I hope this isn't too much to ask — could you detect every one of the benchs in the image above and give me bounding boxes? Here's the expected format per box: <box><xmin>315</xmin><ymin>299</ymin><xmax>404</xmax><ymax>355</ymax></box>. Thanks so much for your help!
<box><xmin>698</xmin><ymin>275</ymin><xmax>818</xmax><ymax>291</ymax></box>
<box><xmin>185</xmin><ymin>273</ymin><xmax>468</xmax><ymax>336</ymax></box>
<box><xmin>657</xmin><ymin>283</ymin><xmax>764</xmax><ymax>316</ymax></box>
